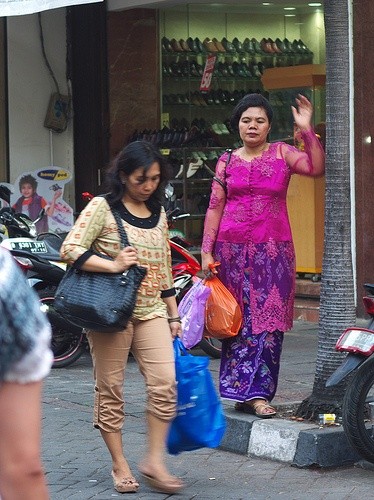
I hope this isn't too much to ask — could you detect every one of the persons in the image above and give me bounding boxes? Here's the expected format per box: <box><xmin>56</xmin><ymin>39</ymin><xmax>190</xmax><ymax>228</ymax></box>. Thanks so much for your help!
<box><xmin>60</xmin><ymin>140</ymin><xmax>189</xmax><ymax>494</ymax></box>
<box><xmin>202</xmin><ymin>93</ymin><xmax>325</xmax><ymax>418</ymax></box>
<box><xmin>10</xmin><ymin>174</ymin><xmax>62</xmax><ymax>236</ymax></box>
<box><xmin>0</xmin><ymin>247</ymin><xmax>55</xmax><ymax>500</ymax></box>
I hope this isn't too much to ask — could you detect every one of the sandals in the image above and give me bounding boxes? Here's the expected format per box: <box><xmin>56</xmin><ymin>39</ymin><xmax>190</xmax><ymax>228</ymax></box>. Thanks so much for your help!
<box><xmin>235</xmin><ymin>400</ymin><xmax>276</xmax><ymax>418</ymax></box>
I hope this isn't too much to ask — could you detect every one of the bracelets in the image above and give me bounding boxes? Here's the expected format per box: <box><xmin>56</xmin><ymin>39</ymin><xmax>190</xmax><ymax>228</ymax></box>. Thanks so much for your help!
<box><xmin>168</xmin><ymin>317</ymin><xmax>182</xmax><ymax>323</ymax></box>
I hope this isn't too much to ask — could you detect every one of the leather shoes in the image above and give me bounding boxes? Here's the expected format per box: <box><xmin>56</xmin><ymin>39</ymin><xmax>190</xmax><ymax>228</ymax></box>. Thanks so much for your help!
<box><xmin>161</xmin><ymin>36</ymin><xmax>310</xmax><ymax>53</ymax></box>
<box><xmin>163</xmin><ymin>89</ymin><xmax>294</xmax><ymax>108</ymax></box>
<box><xmin>163</xmin><ymin>61</ymin><xmax>268</xmax><ymax>78</ymax></box>
<box><xmin>130</xmin><ymin>125</ymin><xmax>200</xmax><ymax>147</ymax></box>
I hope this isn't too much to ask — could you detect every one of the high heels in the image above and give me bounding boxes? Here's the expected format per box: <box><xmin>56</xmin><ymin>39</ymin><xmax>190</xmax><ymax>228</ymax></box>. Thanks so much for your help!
<box><xmin>137</xmin><ymin>467</ymin><xmax>187</xmax><ymax>494</ymax></box>
<box><xmin>110</xmin><ymin>471</ymin><xmax>139</xmax><ymax>493</ymax></box>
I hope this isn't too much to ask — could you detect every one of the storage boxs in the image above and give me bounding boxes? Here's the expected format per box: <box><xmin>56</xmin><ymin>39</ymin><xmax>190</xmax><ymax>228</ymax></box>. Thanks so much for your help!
<box><xmin>262</xmin><ymin>63</ymin><xmax>325</xmax><ymax>175</ymax></box>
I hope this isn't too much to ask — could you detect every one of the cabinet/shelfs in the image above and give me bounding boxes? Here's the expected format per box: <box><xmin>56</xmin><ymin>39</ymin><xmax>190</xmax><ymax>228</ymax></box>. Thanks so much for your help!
<box><xmin>162</xmin><ymin>49</ymin><xmax>314</xmax><ymax>256</ymax></box>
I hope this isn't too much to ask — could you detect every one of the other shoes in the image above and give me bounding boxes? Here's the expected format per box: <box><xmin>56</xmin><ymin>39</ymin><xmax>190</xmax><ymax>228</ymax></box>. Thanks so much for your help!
<box><xmin>187</xmin><ymin>156</ymin><xmax>204</xmax><ymax>178</ymax></box>
<box><xmin>167</xmin><ymin>117</ymin><xmax>239</xmax><ymax>134</ymax></box>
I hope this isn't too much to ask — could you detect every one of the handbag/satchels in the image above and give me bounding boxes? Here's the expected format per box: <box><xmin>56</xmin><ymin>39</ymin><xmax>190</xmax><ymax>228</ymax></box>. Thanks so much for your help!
<box><xmin>48</xmin><ymin>194</ymin><xmax>147</xmax><ymax>332</ymax></box>
<box><xmin>204</xmin><ymin>261</ymin><xmax>243</xmax><ymax>340</ymax></box>
<box><xmin>165</xmin><ymin>337</ymin><xmax>227</xmax><ymax>454</ymax></box>
<box><xmin>178</xmin><ymin>277</ymin><xmax>211</xmax><ymax>349</ymax></box>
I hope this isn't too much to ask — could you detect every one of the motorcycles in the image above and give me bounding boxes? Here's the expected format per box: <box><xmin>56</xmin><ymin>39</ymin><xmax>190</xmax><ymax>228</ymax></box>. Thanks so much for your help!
<box><xmin>0</xmin><ymin>185</ymin><xmax>223</xmax><ymax>369</ymax></box>
<box><xmin>326</xmin><ymin>284</ymin><xmax>374</xmax><ymax>463</ymax></box>
